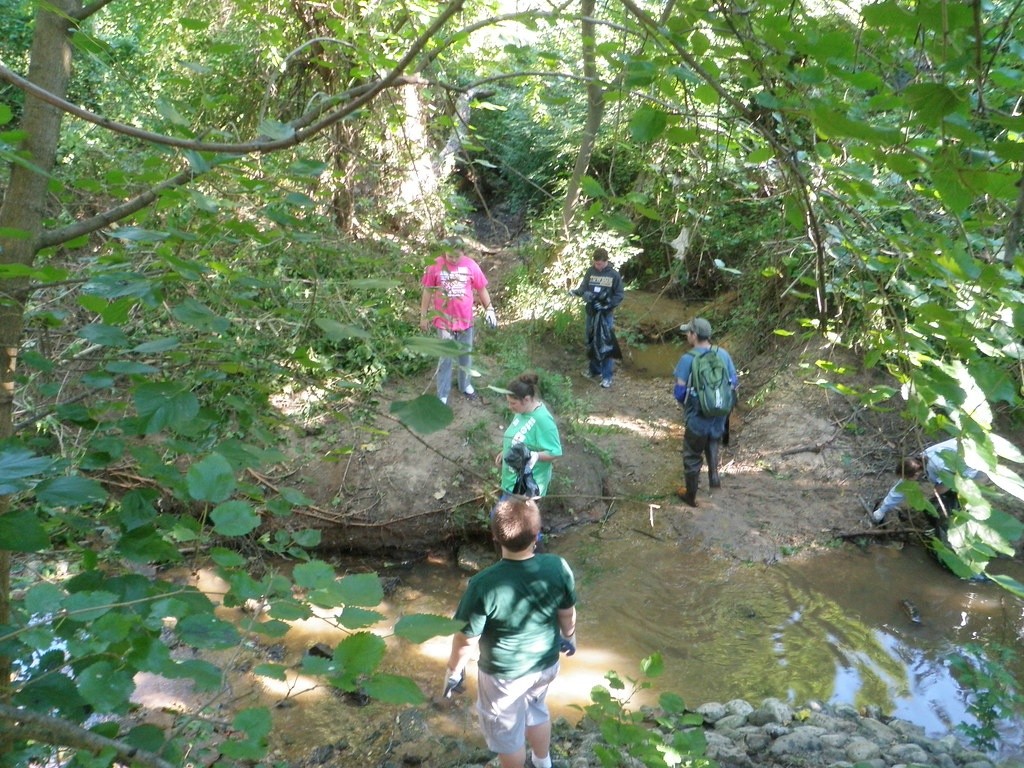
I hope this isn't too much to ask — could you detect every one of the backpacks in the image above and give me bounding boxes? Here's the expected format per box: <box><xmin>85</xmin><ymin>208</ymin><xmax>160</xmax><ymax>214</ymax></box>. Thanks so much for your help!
<box><xmin>685</xmin><ymin>345</ymin><xmax>735</xmax><ymax>416</ymax></box>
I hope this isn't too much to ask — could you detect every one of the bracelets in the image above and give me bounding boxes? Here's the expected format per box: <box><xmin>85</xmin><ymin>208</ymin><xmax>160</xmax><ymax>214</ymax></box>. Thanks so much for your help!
<box><xmin>421</xmin><ymin>313</ymin><xmax>428</xmax><ymax>319</ymax></box>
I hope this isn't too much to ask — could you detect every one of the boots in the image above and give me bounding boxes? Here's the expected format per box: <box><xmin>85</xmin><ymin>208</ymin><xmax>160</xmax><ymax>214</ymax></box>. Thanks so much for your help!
<box><xmin>704</xmin><ymin>435</ymin><xmax>720</xmax><ymax>488</ymax></box>
<box><xmin>674</xmin><ymin>424</ymin><xmax>705</xmax><ymax>507</ymax></box>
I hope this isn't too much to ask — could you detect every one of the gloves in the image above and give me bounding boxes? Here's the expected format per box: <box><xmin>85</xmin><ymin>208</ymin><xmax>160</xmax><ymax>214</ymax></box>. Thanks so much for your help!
<box><xmin>485</xmin><ymin>307</ymin><xmax>496</xmax><ymax>329</ymax></box>
<box><xmin>570</xmin><ymin>290</ymin><xmax>582</xmax><ymax>298</ymax></box>
<box><xmin>593</xmin><ymin>302</ymin><xmax>608</xmax><ymax>310</ymax></box>
<box><xmin>872</xmin><ymin>508</ymin><xmax>886</xmax><ymax>524</ymax></box>
<box><xmin>526</xmin><ymin>451</ymin><xmax>538</xmax><ymax>469</ymax></box>
<box><xmin>559</xmin><ymin>626</ymin><xmax>576</xmax><ymax>656</ymax></box>
<box><xmin>441</xmin><ymin>661</ymin><xmax>466</xmax><ymax>699</ymax></box>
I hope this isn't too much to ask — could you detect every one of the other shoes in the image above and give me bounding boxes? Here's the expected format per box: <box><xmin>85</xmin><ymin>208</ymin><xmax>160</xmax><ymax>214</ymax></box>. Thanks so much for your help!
<box><xmin>440</xmin><ymin>397</ymin><xmax>447</xmax><ymax>404</ymax></box>
<box><xmin>465</xmin><ymin>384</ymin><xmax>474</xmax><ymax>394</ymax></box>
<box><xmin>599</xmin><ymin>378</ymin><xmax>612</xmax><ymax>388</ymax></box>
<box><xmin>581</xmin><ymin>370</ymin><xmax>593</xmax><ymax>378</ymax></box>
<box><xmin>524</xmin><ymin>749</ymin><xmax>554</xmax><ymax>768</ymax></box>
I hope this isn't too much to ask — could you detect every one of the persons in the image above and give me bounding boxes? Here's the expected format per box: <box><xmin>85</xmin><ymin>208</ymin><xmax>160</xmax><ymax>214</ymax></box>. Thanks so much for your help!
<box><xmin>491</xmin><ymin>373</ymin><xmax>563</xmax><ymax>553</ymax></box>
<box><xmin>570</xmin><ymin>248</ymin><xmax>625</xmax><ymax>389</ymax></box>
<box><xmin>871</xmin><ymin>433</ymin><xmax>996</xmax><ymax>584</ymax></box>
<box><xmin>673</xmin><ymin>317</ymin><xmax>738</xmax><ymax>509</ymax></box>
<box><xmin>442</xmin><ymin>494</ymin><xmax>577</xmax><ymax>768</ymax></box>
<box><xmin>419</xmin><ymin>236</ymin><xmax>499</xmax><ymax>407</ymax></box>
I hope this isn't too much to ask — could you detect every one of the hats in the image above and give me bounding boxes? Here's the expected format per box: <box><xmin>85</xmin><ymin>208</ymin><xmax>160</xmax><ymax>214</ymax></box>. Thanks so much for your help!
<box><xmin>679</xmin><ymin>317</ymin><xmax>711</xmax><ymax>337</ymax></box>
